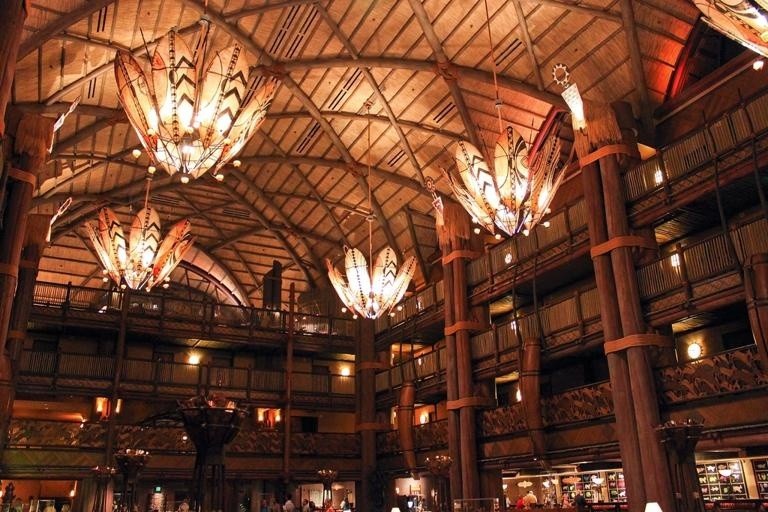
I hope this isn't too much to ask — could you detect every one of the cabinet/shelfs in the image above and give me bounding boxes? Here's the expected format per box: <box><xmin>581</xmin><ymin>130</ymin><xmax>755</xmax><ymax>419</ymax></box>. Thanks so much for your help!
<box><xmin>556</xmin><ymin>456</ymin><xmax>768</xmax><ymax>503</ymax></box>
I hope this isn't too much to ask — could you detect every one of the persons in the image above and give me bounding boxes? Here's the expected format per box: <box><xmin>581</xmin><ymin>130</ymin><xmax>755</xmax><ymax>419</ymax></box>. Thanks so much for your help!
<box><xmin>504</xmin><ymin>491</ymin><xmax>586</xmax><ymax>512</ymax></box>
<box><xmin>112</xmin><ymin>498</ymin><xmax>195</xmax><ymax>512</ymax></box>
<box><xmin>0</xmin><ymin>496</ymin><xmax>81</xmax><ymax>511</ymax></box>
<box><xmin>395</xmin><ymin>490</ymin><xmax>451</xmax><ymax>512</ymax></box>
<box><xmin>261</xmin><ymin>492</ymin><xmax>352</xmax><ymax>512</ymax></box>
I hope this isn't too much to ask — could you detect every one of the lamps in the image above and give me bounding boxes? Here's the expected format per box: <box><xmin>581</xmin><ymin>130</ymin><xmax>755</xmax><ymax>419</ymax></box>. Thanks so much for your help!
<box><xmin>86</xmin><ymin>165</ymin><xmax>197</xmax><ymax>294</ymax></box>
<box><xmin>113</xmin><ymin>0</ymin><xmax>286</xmax><ymax>184</ymax></box>
<box><xmin>442</xmin><ymin>0</ymin><xmax>573</xmax><ymax>264</ymax></box>
<box><xmin>327</xmin><ymin>101</ymin><xmax>417</xmax><ymax>320</ymax></box>
